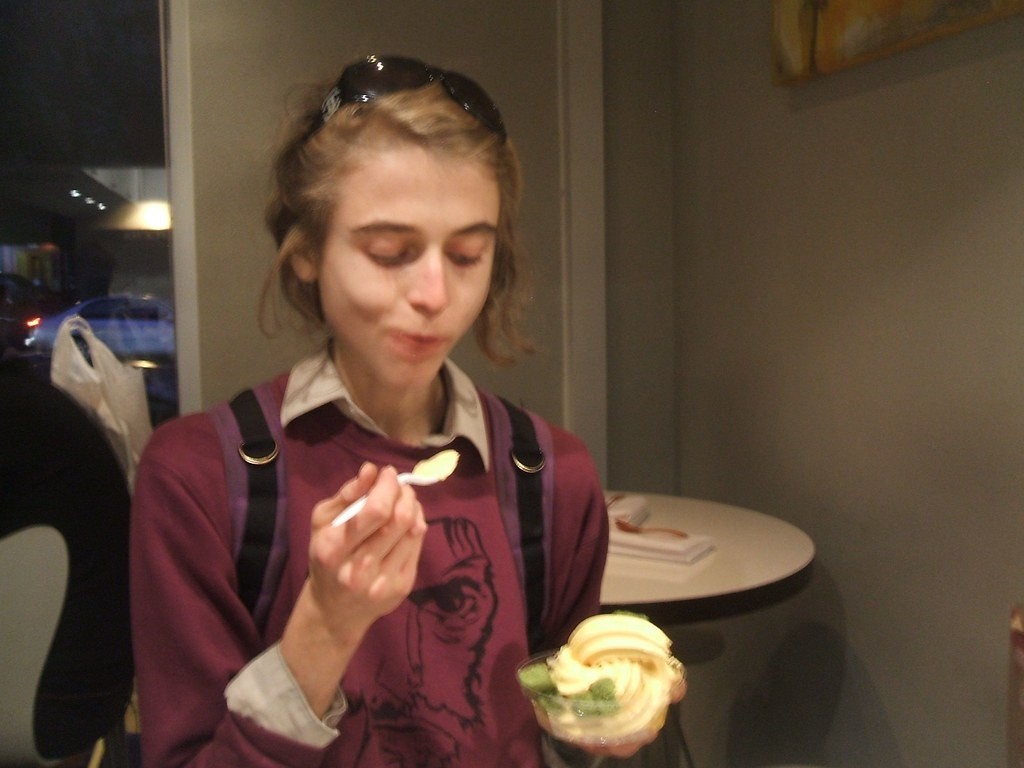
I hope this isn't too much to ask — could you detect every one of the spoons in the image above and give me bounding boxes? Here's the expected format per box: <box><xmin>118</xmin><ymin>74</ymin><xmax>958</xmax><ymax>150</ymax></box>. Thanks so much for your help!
<box><xmin>331</xmin><ymin>451</ymin><xmax>457</xmax><ymax>526</ymax></box>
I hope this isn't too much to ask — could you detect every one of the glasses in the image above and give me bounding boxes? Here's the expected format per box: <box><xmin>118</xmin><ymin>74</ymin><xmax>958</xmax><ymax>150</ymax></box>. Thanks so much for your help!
<box><xmin>310</xmin><ymin>53</ymin><xmax>507</xmax><ymax>147</ymax></box>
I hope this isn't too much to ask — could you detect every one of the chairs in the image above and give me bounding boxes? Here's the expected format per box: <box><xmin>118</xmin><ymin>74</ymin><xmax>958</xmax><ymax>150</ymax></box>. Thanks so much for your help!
<box><xmin>0</xmin><ymin>525</ymin><xmax>112</xmax><ymax>768</ymax></box>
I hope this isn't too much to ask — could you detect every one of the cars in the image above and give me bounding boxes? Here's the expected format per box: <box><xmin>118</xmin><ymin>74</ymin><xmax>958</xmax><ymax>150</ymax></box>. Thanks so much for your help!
<box><xmin>22</xmin><ymin>291</ymin><xmax>174</xmax><ymax>364</ymax></box>
<box><xmin>0</xmin><ymin>269</ymin><xmax>83</xmax><ymax>350</ymax></box>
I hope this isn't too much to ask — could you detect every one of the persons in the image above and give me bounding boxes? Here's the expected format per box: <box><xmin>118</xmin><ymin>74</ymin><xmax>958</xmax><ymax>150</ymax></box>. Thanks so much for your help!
<box><xmin>131</xmin><ymin>55</ymin><xmax>687</xmax><ymax>768</ymax></box>
<box><xmin>0</xmin><ymin>349</ymin><xmax>132</xmax><ymax>762</ymax></box>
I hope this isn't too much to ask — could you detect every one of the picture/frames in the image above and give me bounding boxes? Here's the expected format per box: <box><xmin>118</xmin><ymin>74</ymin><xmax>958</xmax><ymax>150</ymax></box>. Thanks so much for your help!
<box><xmin>772</xmin><ymin>0</ymin><xmax>1024</xmax><ymax>88</ymax></box>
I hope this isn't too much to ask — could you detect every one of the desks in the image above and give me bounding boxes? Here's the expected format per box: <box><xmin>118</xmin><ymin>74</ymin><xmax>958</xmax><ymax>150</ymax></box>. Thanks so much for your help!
<box><xmin>540</xmin><ymin>490</ymin><xmax>814</xmax><ymax>768</ymax></box>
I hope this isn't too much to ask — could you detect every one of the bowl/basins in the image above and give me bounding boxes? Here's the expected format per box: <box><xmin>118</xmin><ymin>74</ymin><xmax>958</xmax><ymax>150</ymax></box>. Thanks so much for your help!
<box><xmin>516</xmin><ymin>652</ymin><xmax>685</xmax><ymax>748</ymax></box>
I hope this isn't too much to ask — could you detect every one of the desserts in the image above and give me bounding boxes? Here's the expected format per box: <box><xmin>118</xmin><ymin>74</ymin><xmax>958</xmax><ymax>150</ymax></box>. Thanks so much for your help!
<box><xmin>520</xmin><ymin>611</ymin><xmax>673</xmax><ymax>740</ymax></box>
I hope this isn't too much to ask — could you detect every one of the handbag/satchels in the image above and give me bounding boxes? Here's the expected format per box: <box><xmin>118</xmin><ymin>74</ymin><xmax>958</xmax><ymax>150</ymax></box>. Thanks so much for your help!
<box><xmin>49</xmin><ymin>316</ymin><xmax>153</xmax><ymax>498</ymax></box>
